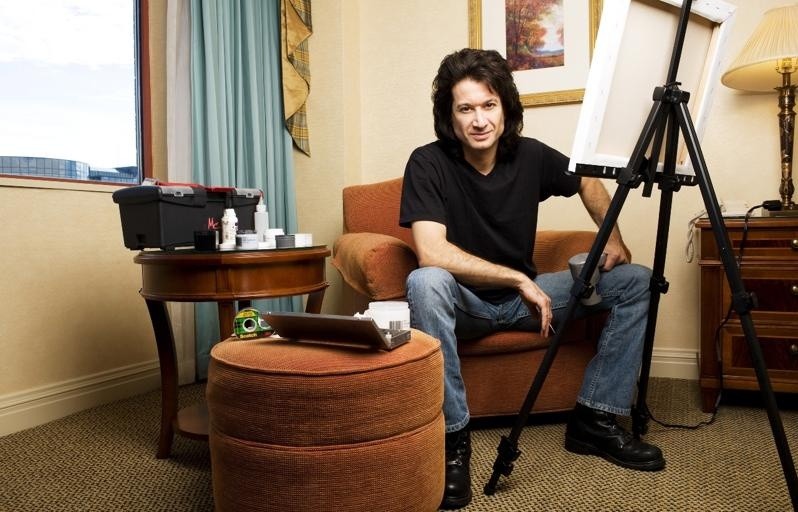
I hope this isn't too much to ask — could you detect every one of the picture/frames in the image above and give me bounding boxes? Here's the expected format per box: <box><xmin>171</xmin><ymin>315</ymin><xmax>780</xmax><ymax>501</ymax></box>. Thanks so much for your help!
<box><xmin>468</xmin><ymin>0</ymin><xmax>603</xmax><ymax>106</ymax></box>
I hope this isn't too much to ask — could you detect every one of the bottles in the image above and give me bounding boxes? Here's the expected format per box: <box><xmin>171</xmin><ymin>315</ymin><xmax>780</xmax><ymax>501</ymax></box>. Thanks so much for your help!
<box><xmin>222</xmin><ymin>208</ymin><xmax>238</xmax><ymax>243</ymax></box>
<box><xmin>255</xmin><ymin>194</ymin><xmax>268</xmax><ymax>236</ymax></box>
<box><xmin>369</xmin><ymin>301</ymin><xmax>410</xmax><ymax>331</ymax></box>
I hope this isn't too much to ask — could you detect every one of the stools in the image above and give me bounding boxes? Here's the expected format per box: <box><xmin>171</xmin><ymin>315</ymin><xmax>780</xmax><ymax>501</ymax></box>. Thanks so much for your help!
<box><xmin>207</xmin><ymin>329</ymin><xmax>445</xmax><ymax>512</ymax></box>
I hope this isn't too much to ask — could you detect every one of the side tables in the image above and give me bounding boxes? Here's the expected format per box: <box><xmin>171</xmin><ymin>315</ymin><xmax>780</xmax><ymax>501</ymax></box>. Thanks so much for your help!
<box><xmin>133</xmin><ymin>245</ymin><xmax>332</xmax><ymax>460</ymax></box>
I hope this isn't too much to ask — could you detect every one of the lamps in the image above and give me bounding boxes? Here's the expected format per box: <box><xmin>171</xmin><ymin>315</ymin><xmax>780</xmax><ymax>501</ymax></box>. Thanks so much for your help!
<box><xmin>721</xmin><ymin>5</ymin><xmax>797</xmax><ymax>218</ymax></box>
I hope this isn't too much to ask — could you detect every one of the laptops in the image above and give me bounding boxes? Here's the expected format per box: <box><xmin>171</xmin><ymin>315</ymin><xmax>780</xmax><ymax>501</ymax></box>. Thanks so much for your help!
<box><xmin>260</xmin><ymin>311</ymin><xmax>411</xmax><ymax>351</ymax></box>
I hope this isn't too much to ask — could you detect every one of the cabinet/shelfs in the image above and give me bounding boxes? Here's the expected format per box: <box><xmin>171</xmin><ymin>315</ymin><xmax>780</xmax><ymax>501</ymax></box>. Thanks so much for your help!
<box><xmin>694</xmin><ymin>219</ymin><xmax>797</xmax><ymax>413</ymax></box>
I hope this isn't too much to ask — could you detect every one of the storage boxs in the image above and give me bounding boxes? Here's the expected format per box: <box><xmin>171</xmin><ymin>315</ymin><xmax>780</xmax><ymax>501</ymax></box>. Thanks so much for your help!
<box><xmin>112</xmin><ymin>180</ymin><xmax>264</xmax><ymax>251</ymax></box>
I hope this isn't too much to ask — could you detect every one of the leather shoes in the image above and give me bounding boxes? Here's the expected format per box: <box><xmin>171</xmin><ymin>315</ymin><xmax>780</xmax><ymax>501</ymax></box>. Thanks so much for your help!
<box><xmin>564</xmin><ymin>403</ymin><xmax>664</xmax><ymax>469</ymax></box>
<box><xmin>440</xmin><ymin>426</ymin><xmax>471</xmax><ymax>509</ymax></box>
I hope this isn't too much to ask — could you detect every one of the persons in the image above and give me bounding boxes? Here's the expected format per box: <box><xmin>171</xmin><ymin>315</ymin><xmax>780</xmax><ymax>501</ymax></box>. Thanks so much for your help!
<box><xmin>398</xmin><ymin>47</ymin><xmax>666</xmax><ymax>510</ymax></box>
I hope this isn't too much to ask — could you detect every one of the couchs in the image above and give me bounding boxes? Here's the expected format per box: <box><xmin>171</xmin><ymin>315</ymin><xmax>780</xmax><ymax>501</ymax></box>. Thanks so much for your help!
<box><xmin>329</xmin><ymin>178</ymin><xmax>598</xmax><ymax>419</ymax></box>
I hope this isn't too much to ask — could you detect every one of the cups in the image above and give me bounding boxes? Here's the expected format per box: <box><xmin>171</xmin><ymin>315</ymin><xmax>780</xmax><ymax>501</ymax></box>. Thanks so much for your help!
<box><xmin>569</xmin><ymin>252</ymin><xmax>607</xmax><ymax>304</ymax></box>
<box><xmin>274</xmin><ymin>234</ymin><xmax>295</xmax><ymax>248</ymax></box>
